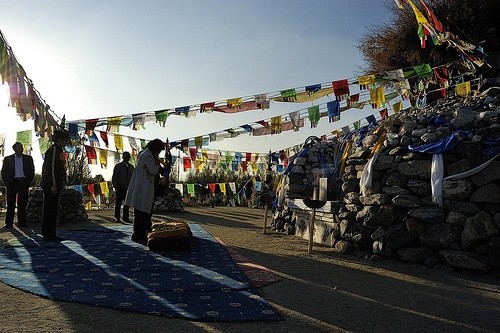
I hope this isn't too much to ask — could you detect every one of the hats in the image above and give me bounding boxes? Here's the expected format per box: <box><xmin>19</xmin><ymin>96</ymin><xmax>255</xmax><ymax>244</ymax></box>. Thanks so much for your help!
<box><xmin>54</xmin><ymin>129</ymin><xmax>69</xmax><ymax>138</ymax></box>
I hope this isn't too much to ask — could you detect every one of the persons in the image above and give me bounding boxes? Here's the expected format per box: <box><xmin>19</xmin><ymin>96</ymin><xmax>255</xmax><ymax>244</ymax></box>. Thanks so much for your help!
<box><xmin>0</xmin><ymin>142</ymin><xmax>35</xmax><ymax>228</ymax></box>
<box><xmin>40</xmin><ymin>130</ymin><xmax>70</xmax><ymax>236</ymax></box>
<box><xmin>126</xmin><ymin>139</ymin><xmax>171</xmax><ymax>243</ymax></box>
<box><xmin>112</xmin><ymin>151</ymin><xmax>134</xmax><ymax>223</ymax></box>
<box><xmin>146</xmin><ymin>153</ymin><xmax>161</xmax><ymax>232</ymax></box>
